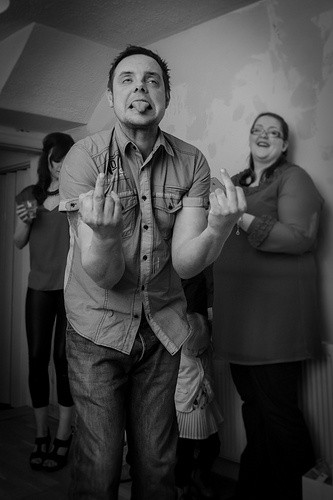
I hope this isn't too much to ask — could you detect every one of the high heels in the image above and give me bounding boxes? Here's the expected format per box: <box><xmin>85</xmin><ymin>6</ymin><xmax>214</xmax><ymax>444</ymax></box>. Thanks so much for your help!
<box><xmin>28</xmin><ymin>426</ymin><xmax>52</xmax><ymax>471</ymax></box>
<box><xmin>43</xmin><ymin>426</ymin><xmax>74</xmax><ymax>472</ymax></box>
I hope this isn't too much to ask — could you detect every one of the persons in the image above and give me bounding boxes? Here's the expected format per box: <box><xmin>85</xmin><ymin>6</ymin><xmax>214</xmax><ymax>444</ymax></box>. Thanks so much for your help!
<box><xmin>59</xmin><ymin>47</ymin><xmax>247</xmax><ymax>500</ymax></box>
<box><xmin>14</xmin><ymin>133</ymin><xmax>75</xmax><ymax>474</ymax></box>
<box><xmin>174</xmin><ymin>313</ymin><xmax>224</xmax><ymax>500</ymax></box>
<box><xmin>212</xmin><ymin>113</ymin><xmax>324</xmax><ymax>500</ymax></box>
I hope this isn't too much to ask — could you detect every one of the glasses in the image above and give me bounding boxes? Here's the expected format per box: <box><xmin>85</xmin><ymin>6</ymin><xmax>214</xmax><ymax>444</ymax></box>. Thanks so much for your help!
<box><xmin>250</xmin><ymin>127</ymin><xmax>284</xmax><ymax>140</ymax></box>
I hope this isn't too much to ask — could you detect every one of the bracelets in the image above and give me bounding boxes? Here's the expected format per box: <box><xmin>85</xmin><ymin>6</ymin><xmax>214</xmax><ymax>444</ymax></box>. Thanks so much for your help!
<box><xmin>237</xmin><ymin>216</ymin><xmax>244</xmax><ymax>236</ymax></box>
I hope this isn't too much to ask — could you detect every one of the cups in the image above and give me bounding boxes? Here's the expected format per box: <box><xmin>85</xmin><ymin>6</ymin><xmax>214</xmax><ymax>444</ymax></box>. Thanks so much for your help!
<box><xmin>24</xmin><ymin>199</ymin><xmax>36</xmax><ymax>219</ymax></box>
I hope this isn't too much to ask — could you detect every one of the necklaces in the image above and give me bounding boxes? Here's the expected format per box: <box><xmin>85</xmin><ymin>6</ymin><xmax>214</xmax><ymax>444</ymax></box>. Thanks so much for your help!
<box><xmin>46</xmin><ymin>188</ymin><xmax>59</xmax><ymax>195</ymax></box>
